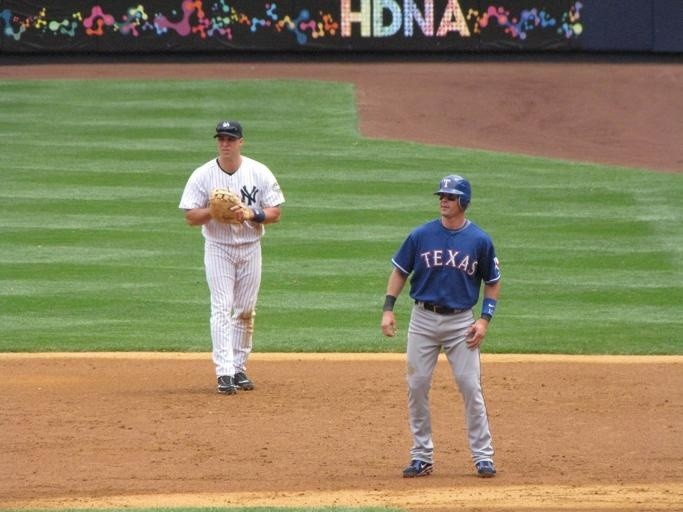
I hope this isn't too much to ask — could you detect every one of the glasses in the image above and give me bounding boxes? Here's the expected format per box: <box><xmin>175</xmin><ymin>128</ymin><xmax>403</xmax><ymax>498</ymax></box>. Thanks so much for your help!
<box><xmin>439</xmin><ymin>193</ymin><xmax>456</xmax><ymax>201</ymax></box>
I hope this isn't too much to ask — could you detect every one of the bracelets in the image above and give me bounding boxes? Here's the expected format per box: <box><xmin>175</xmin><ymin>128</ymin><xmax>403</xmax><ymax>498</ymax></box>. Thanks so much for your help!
<box><xmin>383</xmin><ymin>295</ymin><xmax>396</xmax><ymax>311</ymax></box>
<box><xmin>481</xmin><ymin>299</ymin><xmax>496</xmax><ymax>318</ymax></box>
<box><xmin>251</xmin><ymin>209</ymin><xmax>265</xmax><ymax>223</ymax></box>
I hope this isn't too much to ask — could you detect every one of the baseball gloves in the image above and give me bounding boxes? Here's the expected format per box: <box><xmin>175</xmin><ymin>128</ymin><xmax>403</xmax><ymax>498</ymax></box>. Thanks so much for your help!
<box><xmin>209</xmin><ymin>188</ymin><xmax>244</xmax><ymax>224</ymax></box>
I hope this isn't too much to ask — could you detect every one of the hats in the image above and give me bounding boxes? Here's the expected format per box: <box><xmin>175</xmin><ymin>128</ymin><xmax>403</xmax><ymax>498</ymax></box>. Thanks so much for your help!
<box><xmin>214</xmin><ymin>120</ymin><xmax>242</xmax><ymax>139</ymax></box>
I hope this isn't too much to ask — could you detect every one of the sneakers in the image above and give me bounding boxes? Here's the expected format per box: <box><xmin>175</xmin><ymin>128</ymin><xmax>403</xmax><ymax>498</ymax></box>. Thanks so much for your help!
<box><xmin>403</xmin><ymin>460</ymin><xmax>433</xmax><ymax>477</ymax></box>
<box><xmin>476</xmin><ymin>461</ymin><xmax>496</xmax><ymax>477</ymax></box>
<box><xmin>234</xmin><ymin>372</ymin><xmax>253</xmax><ymax>390</ymax></box>
<box><xmin>217</xmin><ymin>376</ymin><xmax>238</xmax><ymax>395</ymax></box>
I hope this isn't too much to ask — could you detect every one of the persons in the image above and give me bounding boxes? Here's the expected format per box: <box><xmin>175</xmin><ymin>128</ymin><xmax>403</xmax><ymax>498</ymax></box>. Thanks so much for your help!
<box><xmin>178</xmin><ymin>122</ymin><xmax>287</xmax><ymax>394</ymax></box>
<box><xmin>381</xmin><ymin>175</ymin><xmax>501</xmax><ymax>479</ymax></box>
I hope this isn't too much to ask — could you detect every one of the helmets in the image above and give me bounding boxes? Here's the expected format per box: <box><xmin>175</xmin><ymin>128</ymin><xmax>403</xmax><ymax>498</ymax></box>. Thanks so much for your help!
<box><xmin>434</xmin><ymin>175</ymin><xmax>471</xmax><ymax>210</ymax></box>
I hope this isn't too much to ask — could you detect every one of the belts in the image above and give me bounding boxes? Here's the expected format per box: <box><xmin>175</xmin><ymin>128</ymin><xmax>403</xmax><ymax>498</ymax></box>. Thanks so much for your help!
<box><xmin>415</xmin><ymin>300</ymin><xmax>465</xmax><ymax>314</ymax></box>
